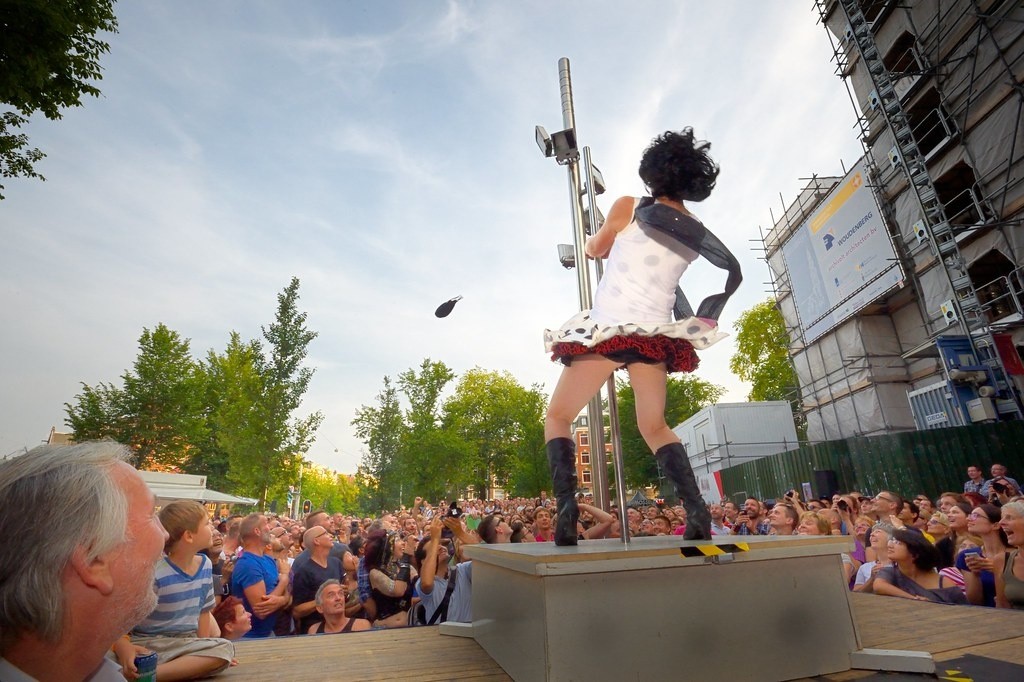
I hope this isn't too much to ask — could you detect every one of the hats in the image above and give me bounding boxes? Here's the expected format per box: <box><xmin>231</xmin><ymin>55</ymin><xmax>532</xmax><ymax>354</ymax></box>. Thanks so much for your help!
<box><xmin>489</xmin><ymin>498</ymin><xmax>495</xmax><ymax>502</ymax></box>
<box><xmin>858</xmin><ymin>495</ymin><xmax>874</xmax><ymax>501</ymax></box>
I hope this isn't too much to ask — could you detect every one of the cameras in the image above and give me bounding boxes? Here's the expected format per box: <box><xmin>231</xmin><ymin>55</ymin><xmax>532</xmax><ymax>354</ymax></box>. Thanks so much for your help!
<box><xmin>836</xmin><ymin>500</ymin><xmax>852</xmax><ymax>514</ymax></box>
<box><xmin>228</xmin><ymin>553</ymin><xmax>237</xmax><ymax>562</ymax></box>
<box><xmin>992</xmin><ymin>482</ymin><xmax>1009</xmax><ymax>493</ymax></box>
<box><xmin>441</xmin><ymin>501</ymin><xmax>463</xmax><ymax>537</ymax></box>
<box><xmin>786</xmin><ymin>491</ymin><xmax>793</xmax><ymax>501</ymax></box>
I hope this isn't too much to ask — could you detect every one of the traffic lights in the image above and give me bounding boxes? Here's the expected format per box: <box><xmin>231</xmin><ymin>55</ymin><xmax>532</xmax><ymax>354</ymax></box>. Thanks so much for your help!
<box><xmin>303</xmin><ymin>500</ymin><xmax>311</xmax><ymax>513</ymax></box>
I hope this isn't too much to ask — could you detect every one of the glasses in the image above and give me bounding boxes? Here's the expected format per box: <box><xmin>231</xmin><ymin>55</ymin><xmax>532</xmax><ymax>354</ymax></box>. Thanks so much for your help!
<box><xmin>889</xmin><ymin>537</ymin><xmax>901</xmax><ymax>546</ymax></box>
<box><xmin>928</xmin><ymin>518</ymin><xmax>945</xmax><ymax>527</ymax></box>
<box><xmin>495</xmin><ymin>517</ymin><xmax>505</xmax><ymax>535</ymax></box>
<box><xmin>278</xmin><ymin>531</ymin><xmax>287</xmax><ymax>539</ymax></box>
<box><xmin>875</xmin><ymin>495</ymin><xmax>893</xmax><ymax>502</ymax></box>
<box><xmin>315</xmin><ymin>531</ymin><xmax>328</xmax><ymax>539</ymax></box>
<box><xmin>968</xmin><ymin>512</ymin><xmax>991</xmax><ymax>520</ymax></box>
<box><xmin>808</xmin><ymin>505</ymin><xmax>821</xmax><ymax>509</ymax></box>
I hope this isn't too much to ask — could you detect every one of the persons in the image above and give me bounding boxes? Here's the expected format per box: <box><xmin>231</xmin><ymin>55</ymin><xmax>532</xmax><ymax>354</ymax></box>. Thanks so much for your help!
<box><xmin>105</xmin><ymin>500</ymin><xmax>239</xmax><ymax>682</ymax></box>
<box><xmin>544</xmin><ymin>129</ymin><xmax>743</xmax><ymax>546</ymax></box>
<box><xmin>0</xmin><ymin>442</ymin><xmax>169</xmax><ymax>682</ymax></box>
<box><xmin>132</xmin><ymin>465</ymin><xmax>1024</xmax><ymax>638</ymax></box>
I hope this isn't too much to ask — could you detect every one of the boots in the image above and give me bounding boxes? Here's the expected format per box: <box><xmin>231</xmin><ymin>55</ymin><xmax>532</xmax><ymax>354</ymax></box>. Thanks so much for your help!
<box><xmin>654</xmin><ymin>442</ymin><xmax>714</xmax><ymax>558</ymax></box>
<box><xmin>544</xmin><ymin>437</ymin><xmax>580</xmax><ymax>546</ymax></box>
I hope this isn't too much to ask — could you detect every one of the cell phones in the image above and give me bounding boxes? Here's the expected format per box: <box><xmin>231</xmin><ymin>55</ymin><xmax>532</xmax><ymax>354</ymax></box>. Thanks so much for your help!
<box><xmin>965</xmin><ymin>552</ymin><xmax>981</xmax><ymax>559</ymax></box>
<box><xmin>741</xmin><ymin>509</ymin><xmax>747</xmax><ymax>515</ymax></box>
<box><xmin>351</xmin><ymin>520</ymin><xmax>358</xmax><ymax>534</ymax></box>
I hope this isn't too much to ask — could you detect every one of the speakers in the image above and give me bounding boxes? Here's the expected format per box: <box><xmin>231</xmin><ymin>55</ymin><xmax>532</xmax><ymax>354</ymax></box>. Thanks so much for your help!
<box><xmin>815</xmin><ymin>470</ymin><xmax>839</xmax><ymax>500</ymax></box>
